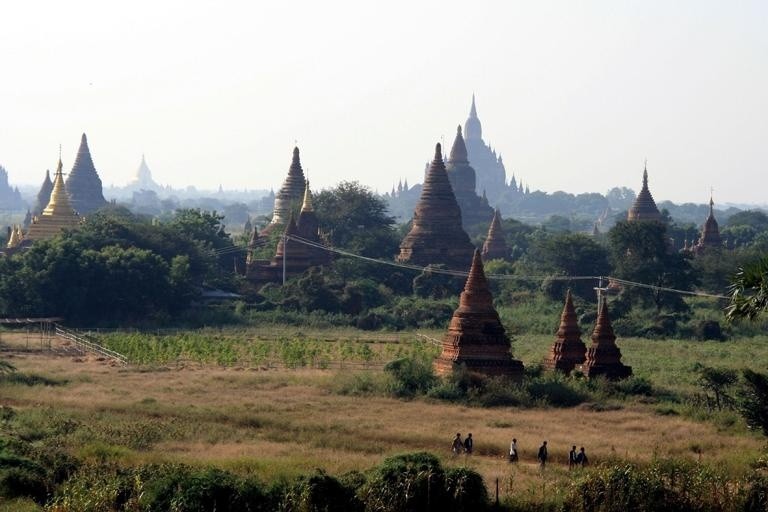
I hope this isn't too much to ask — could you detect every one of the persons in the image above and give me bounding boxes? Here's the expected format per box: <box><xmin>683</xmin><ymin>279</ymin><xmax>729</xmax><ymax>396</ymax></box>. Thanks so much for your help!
<box><xmin>510</xmin><ymin>438</ymin><xmax>518</xmax><ymax>463</ymax></box>
<box><xmin>569</xmin><ymin>446</ymin><xmax>588</xmax><ymax>470</ymax></box>
<box><xmin>538</xmin><ymin>441</ymin><xmax>548</xmax><ymax>465</ymax></box>
<box><xmin>450</xmin><ymin>433</ymin><xmax>473</xmax><ymax>454</ymax></box>
